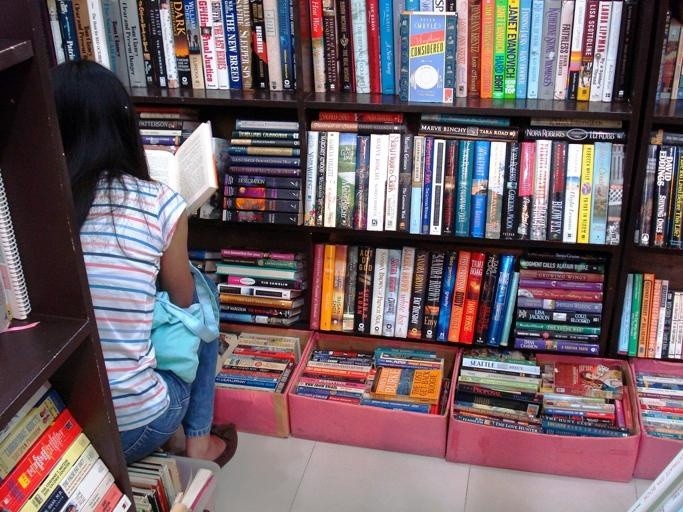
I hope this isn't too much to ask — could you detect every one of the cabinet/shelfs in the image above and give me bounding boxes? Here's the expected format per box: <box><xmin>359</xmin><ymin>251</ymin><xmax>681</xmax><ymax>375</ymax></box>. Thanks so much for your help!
<box><xmin>1</xmin><ymin>2</ymin><xmax>138</xmax><ymax>512</ymax></box>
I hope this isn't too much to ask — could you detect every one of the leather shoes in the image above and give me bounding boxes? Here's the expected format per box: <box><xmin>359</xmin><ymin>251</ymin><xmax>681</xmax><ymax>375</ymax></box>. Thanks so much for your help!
<box><xmin>211</xmin><ymin>422</ymin><xmax>237</xmax><ymax>468</ymax></box>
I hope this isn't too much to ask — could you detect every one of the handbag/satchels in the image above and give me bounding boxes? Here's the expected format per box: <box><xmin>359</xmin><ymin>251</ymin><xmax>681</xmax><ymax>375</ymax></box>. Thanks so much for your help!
<box><xmin>152</xmin><ymin>260</ymin><xmax>220</xmax><ymax>384</ymax></box>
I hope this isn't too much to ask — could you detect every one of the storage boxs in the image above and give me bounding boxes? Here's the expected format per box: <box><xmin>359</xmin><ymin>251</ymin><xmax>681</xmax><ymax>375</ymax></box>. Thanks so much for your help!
<box><xmin>119</xmin><ymin>452</ymin><xmax>224</xmax><ymax>512</ymax></box>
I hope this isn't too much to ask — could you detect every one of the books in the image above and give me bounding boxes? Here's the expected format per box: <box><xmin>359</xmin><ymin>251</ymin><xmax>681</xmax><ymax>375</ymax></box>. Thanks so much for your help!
<box><xmin>46</xmin><ymin>0</ymin><xmax>682</xmax><ymax>512</ymax></box>
<box><xmin>0</xmin><ymin>271</ymin><xmax>12</xmax><ymax>333</ymax></box>
<box><xmin>1</xmin><ymin>385</ymin><xmax>131</xmax><ymax>511</ymax></box>
<box><xmin>0</xmin><ymin>172</ymin><xmax>32</xmax><ymax>324</ymax></box>
